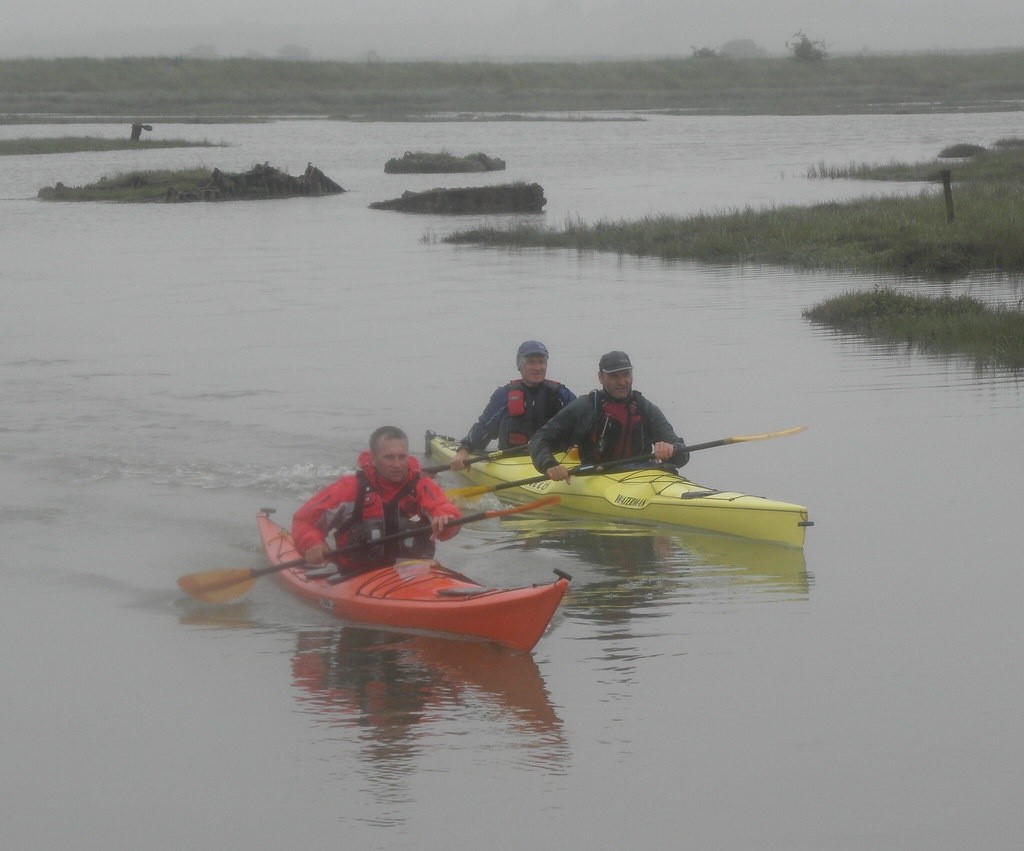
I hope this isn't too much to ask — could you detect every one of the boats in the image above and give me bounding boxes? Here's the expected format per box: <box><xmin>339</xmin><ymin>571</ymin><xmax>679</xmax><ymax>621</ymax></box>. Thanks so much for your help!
<box><xmin>256</xmin><ymin>507</ymin><xmax>573</xmax><ymax>654</ymax></box>
<box><xmin>426</xmin><ymin>430</ymin><xmax>815</xmax><ymax>550</ymax></box>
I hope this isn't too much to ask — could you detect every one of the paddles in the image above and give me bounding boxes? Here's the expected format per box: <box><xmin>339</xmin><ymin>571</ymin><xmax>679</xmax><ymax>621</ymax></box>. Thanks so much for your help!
<box><xmin>176</xmin><ymin>496</ymin><xmax>559</xmax><ymax>605</ymax></box>
<box><xmin>421</xmin><ymin>440</ymin><xmax>529</xmax><ymax>476</ymax></box>
<box><xmin>446</xmin><ymin>422</ymin><xmax>810</xmax><ymax>504</ymax></box>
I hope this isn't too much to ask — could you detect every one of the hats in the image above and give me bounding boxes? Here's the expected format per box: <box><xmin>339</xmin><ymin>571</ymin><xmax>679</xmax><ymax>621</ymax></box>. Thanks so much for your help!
<box><xmin>599</xmin><ymin>351</ymin><xmax>633</xmax><ymax>373</ymax></box>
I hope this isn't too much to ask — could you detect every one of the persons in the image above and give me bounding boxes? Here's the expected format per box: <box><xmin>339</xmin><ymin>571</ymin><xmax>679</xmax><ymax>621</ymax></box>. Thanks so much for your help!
<box><xmin>527</xmin><ymin>351</ymin><xmax>690</xmax><ymax>485</ymax></box>
<box><xmin>291</xmin><ymin>426</ymin><xmax>463</xmax><ymax>580</ymax></box>
<box><xmin>451</xmin><ymin>340</ymin><xmax>578</xmax><ymax>472</ymax></box>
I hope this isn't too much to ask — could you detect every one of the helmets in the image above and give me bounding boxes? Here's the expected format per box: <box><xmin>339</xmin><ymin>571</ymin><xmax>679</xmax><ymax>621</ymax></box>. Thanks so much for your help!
<box><xmin>516</xmin><ymin>340</ymin><xmax>549</xmax><ymax>363</ymax></box>
